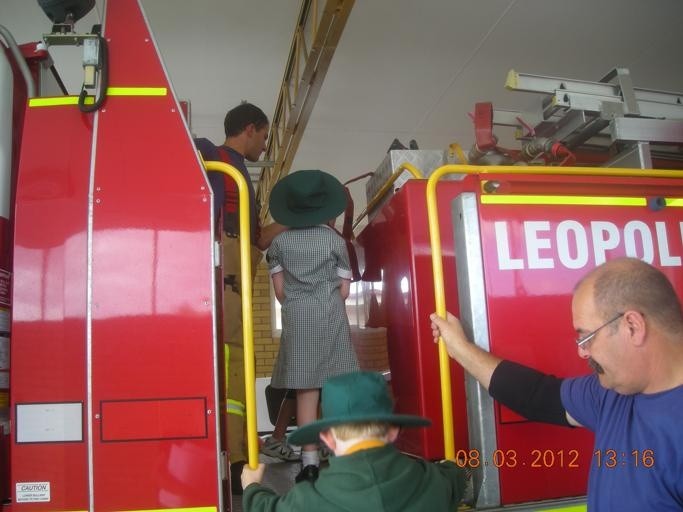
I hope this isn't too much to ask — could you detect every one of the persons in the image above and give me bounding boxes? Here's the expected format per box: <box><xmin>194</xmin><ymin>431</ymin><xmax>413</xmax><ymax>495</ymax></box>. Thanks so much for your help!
<box><xmin>433</xmin><ymin>255</ymin><xmax>683</xmax><ymax>512</ymax></box>
<box><xmin>262</xmin><ymin>389</ymin><xmax>332</xmax><ymax>463</ymax></box>
<box><xmin>240</xmin><ymin>371</ymin><xmax>469</xmax><ymax>512</ymax></box>
<box><xmin>194</xmin><ymin>102</ymin><xmax>290</xmax><ymax>496</ymax></box>
<box><xmin>264</xmin><ymin>169</ymin><xmax>360</xmax><ymax>482</ymax></box>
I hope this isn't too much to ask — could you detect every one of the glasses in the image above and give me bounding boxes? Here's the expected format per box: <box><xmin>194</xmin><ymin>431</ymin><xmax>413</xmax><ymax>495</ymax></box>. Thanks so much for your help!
<box><xmin>576</xmin><ymin>314</ymin><xmax>623</xmax><ymax>350</ymax></box>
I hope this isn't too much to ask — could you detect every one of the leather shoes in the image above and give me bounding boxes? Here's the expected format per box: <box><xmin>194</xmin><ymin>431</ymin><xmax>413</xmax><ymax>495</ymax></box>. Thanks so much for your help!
<box><xmin>296</xmin><ymin>465</ymin><xmax>319</xmax><ymax>482</ymax></box>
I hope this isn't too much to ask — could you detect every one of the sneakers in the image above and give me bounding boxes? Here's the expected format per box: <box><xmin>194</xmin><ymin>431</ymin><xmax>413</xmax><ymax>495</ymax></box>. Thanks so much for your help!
<box><xmin>262</xmin><ymin>436</ymin><xmax>301</xmax><ymax>461</ymax></box>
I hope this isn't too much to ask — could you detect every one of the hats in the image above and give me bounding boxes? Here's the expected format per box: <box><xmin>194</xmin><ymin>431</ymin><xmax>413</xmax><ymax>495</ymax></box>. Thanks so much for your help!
<box><xmin>288</xmin><ymin>372</ymin><xmax>431</xmax><ymax>444</ymax></box>
<box><xmin>268</xmin><ymin>169</ymin><xmax>347</xmax><ymax>226</ymax></box>
